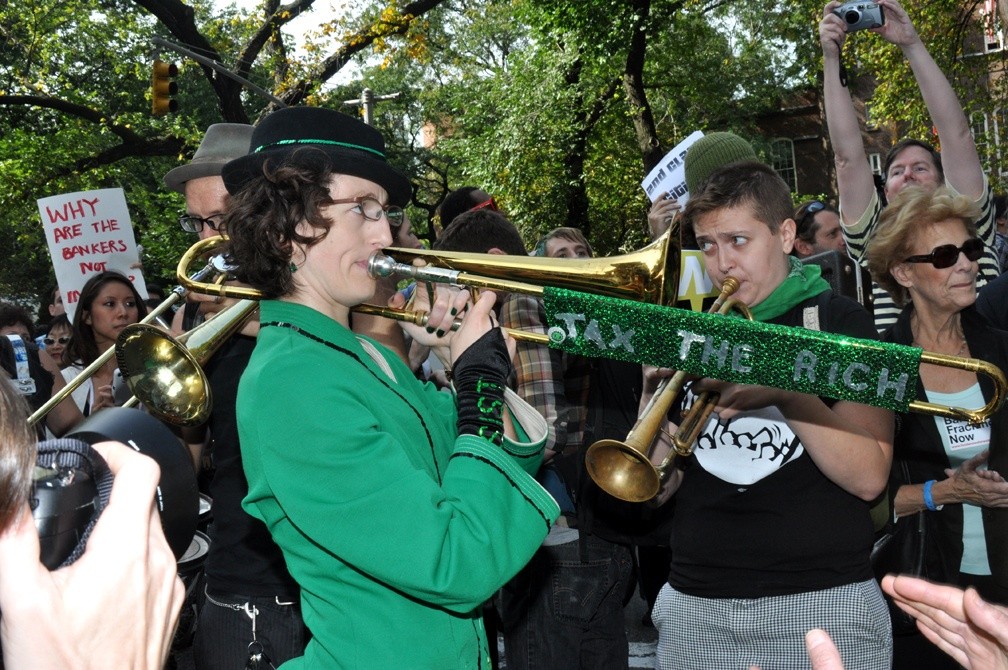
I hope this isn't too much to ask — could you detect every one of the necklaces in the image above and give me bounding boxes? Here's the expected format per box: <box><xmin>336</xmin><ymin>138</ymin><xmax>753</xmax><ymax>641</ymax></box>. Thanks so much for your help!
<box><xmin>910</xmin><ymin>329</ymin><xmax>966</xmax><ymax>356</ymax></box>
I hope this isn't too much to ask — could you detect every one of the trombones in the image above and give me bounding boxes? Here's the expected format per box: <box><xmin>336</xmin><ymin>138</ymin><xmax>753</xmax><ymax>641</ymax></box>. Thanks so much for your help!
<box><xmin>174</xmin><ymin>232</ymin><xmax>1007</xmax><ymax>425</ymax></box>
<box><xmin>16</xmin><ymin>246</ymin><xmax>262</xmax><ymax>428</ymax></box>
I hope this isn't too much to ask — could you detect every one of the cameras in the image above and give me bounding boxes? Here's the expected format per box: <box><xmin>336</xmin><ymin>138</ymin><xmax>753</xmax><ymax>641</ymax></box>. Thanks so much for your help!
<box><xmin>831</xmin><ymin>1</ymin><xmax>885</xmax><ymax>33</ymax></box>
<box><xmin>29</xmin><ymin>408</ymin><xmax>199</xmax><ymax>573</ymax></box>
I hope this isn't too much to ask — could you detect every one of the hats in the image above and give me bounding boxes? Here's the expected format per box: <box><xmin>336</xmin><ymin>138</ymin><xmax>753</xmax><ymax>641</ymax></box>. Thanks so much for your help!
<box><xmin>440</xmin><ymin>187</ymin><xmax>497</xmax><ymax>230</ymax></box>
<box><xmin>222</xmin><ymin>107</ymin><xmax>411</xmax><ymax>207</ymax></box>
<box><xmin>684</xmin><ymin>132</ymin><xmax>759</xmax><ymax>200</ymax></box>
<box><xmin>162</xmin><ymin>123</ymin><xmax>257</xmax><ymax>195</ymax></box>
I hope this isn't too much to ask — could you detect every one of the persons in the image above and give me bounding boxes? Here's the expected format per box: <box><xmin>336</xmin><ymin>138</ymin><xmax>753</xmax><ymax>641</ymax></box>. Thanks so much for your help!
<box><xmin>144</xmin><ymin>283</ymin><xmax>178</xmax><ymax>332</ymax></box>
<box><xmin>992</xmin><ymin>190</ymin><xmax>1008</xmax><ymax>273</ymax></box>
<box><xmin>42</xmin><ymin>271</ymin><xmax>153</xmax><ymax>442</ymax></box>
<box><xmin>818</xmin><ymin>0</ymin><xmax>1002</xmax><ymax>334</ymax></box>
<box><xmin>746</xmin><ymin>575</ymin><xmax>1008</xmax><ymax>670</ymax></box>
<box><xmin>0</xmin><ymin>364</ymin><xmax>187</xmax><ymax>670</ymax></box>
<box><xmin>432</xmin><ymin>209</ymin><xmax>635</xmax><ymax>670</ymax></box>
<box><xmin>168</xmin><ymin>125</ymin><xmax>315</xmax><ymax>669</ymax></box>
<box><xmin>973</xmin><ymin>274</ymin><xmax>1008</xmax><ymax>332</ymax></box>
<box><xmin>684</xmin><ymin>129</ymin><xmax>761</xmax><ymax>197</ymax></box>
<box><xmin>792</xmin><ymin>198</ymin><xmax>851</xmax><ymax>259</ymax></box>
<box><xmin>0</xmin><ymin>334</ymin><xmax>113</xmax><ymax>442</ymax></box>
<box><xmin>0</xmin><ymin>302</ymin><xmax>35</xmax><ymax>345</ymax></box>
<box><xmin>219</xmin><ymin>105</ymin><xmax>564</xmax><ymax>670</ymax></box>
<box><xmin>44</xmin><ymin>312</ymin><xmax>78</xmax><ymax>370</ymax></box>
<box><xmin>350</xmin><ymin>185</ymin><xmax>719</xmax><ymax>396</ymax></box>
<box><xmin>35</xmin><ymin>285</ymin><xmax>67</xmax><ymax>337</ymax></box>
<box><xmin>863</xmin><ymin>183</ymin><xmax>1008</xmax><ymax>670</ymax></box>
<box><xmin>624</xmin><ymin>155</ymin><xmax>897</xmax><ymax>669</ymax></box>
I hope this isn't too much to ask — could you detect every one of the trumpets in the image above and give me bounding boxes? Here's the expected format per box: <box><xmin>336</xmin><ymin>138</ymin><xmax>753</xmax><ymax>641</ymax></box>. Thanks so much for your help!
<box><xmin>583</xmin><ymin>276</ymin><xmax>759</xmax><ymax>504</ymax></box>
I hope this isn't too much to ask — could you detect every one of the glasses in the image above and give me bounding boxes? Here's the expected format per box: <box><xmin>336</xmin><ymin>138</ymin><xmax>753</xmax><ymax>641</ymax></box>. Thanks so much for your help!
<box><xmin>903</xmin><ymin>239</ymin><xmax>984</xmax><ymax>269</ymax></box>
<box><xmin>42</xmin><ymin>335</ymin><xmax>72</xmax><ymax>345</ymax></box>
<box><xmin>318</xmin><ymin>197</ymin><xmax>406</xmax><ymax>228</ymax></box>
<box><xmin>144</xmin><ymin>299</ymin><xmax>162</xmax><ymax>307</ymax></box>
<box><xmin>465</xmin><ymin>196</ymin><xmax>496</xmax><ymax>215</ymax></box>
<box><xmin>794</xmin><ymin>202</ymin><xmax>824</xmax><ymax>228</ymax></box>
<box><xmin>178</xmin><ymin>210</ymin><xmax>231</xmax><ymax>233</ymax></box>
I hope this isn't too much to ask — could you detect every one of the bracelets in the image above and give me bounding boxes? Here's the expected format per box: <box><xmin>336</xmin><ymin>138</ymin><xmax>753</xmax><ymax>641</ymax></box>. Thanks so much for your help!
<box><xmin>923</xmin><ymin>479</ymin><xmax>947</xmax><ymax>512</ymax></box>
